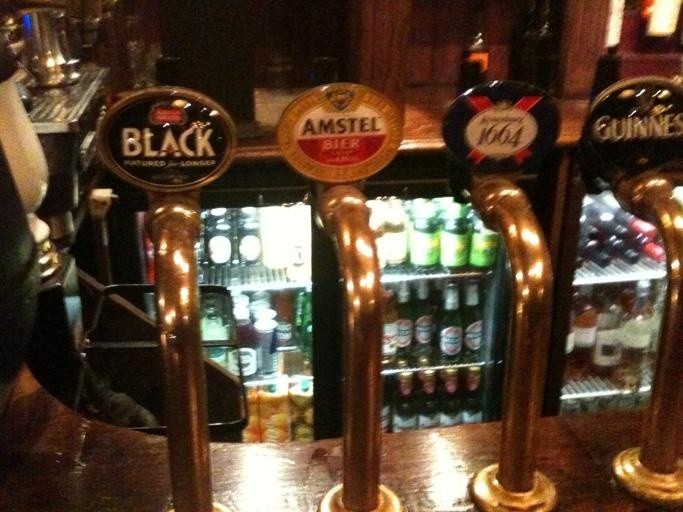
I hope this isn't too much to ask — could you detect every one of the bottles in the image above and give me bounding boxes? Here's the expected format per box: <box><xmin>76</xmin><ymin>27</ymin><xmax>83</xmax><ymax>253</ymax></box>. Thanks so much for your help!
<box><xmin>511</xmin><ymin>0</ymin><xmax>555</xmax><ymax>96</ymax></box>
<box><xmin>458</xmin><ymin>0</ymin><xmax>490</xmax><ymax>96</ymax></box>
<box><xmin>139</xmin><ymin>181</ymin><xmax>669</xmax><ymax>447</ymax></box>
<box><xmin>589</xmin><ymin>47</ymin><xmax>623</xmax><ymax>106</ymax></box>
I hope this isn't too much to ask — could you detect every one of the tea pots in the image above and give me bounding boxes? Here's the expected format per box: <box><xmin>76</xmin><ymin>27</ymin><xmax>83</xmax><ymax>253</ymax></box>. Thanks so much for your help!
<box><xmin>3</xmin><ymin>10</ymin><xmax>83</xmax><ymax>90</ymax></box>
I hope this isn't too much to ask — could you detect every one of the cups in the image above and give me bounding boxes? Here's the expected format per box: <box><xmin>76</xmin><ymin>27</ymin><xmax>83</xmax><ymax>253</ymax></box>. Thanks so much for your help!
<box><xmin>303</xmin><ymin>55</ymin><xmax>341</xmax><ymax>88</ymax></box>
<box><xmin>263</xmin><ymin>44</ymin><xmax>299</xmax><ymax>88</ymax></box>
